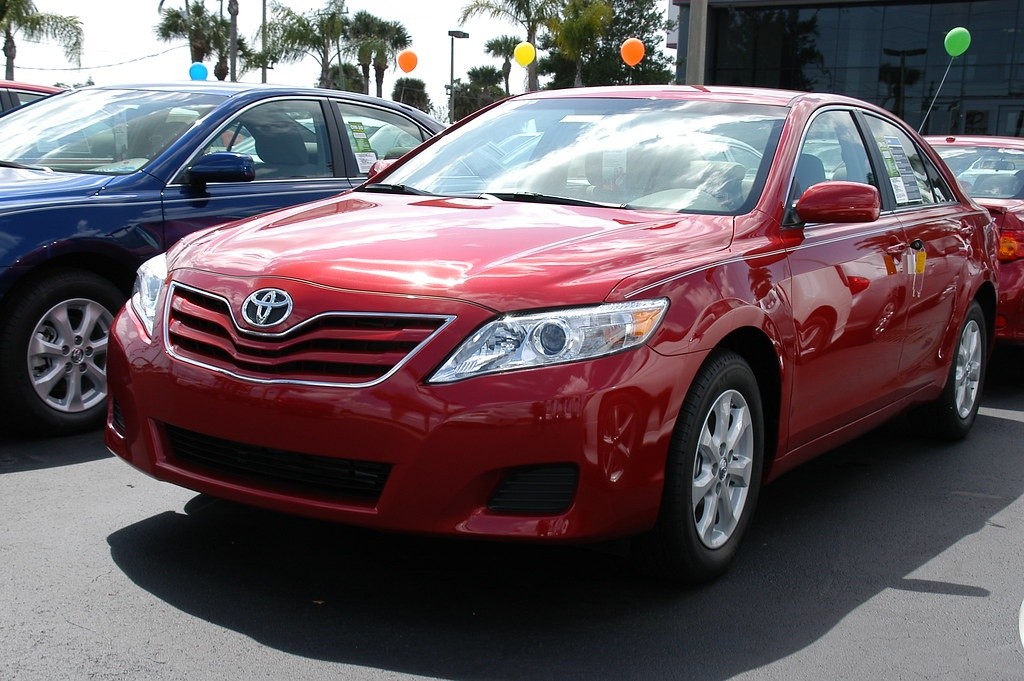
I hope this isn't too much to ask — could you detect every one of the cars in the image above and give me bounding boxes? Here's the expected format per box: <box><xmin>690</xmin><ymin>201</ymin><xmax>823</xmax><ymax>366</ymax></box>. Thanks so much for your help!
<box><xmin>104</xmin><ymin>87</ymin><xmax>1002</xmax><ymax>584</ymax></box>
<box><xmin>0</xmin><ymin>81</ymin><xmax>504</xmax><ymax>435</ymax></box>
<box><xmin>0</xmin><ymin>81</ymin><xmax>897</xmax><ymax>217</ymax></box>
<box><xmin>897</xmin><ymin>135</ymin><xmax>1024</xmax><ymax>348</ymax></box>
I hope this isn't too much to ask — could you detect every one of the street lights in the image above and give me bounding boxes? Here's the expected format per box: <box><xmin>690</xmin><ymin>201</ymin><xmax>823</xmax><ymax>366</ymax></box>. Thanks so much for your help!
<box><xmin>449</xmin><ymin>30</ymin><xmax>469</xmax><ymax>125</ymax></box>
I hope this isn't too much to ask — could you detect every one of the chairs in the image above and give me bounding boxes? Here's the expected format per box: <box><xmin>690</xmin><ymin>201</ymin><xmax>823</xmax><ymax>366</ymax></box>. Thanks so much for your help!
<box><xmin>794</xmin><ymin>154</ymin><xmax>827</xmax><ymax>198</ymax></box>
<box><xmin>257</xmin><ymin>122</ymin><xmax>307</xmax><ymax>182</ymax></box>
<box><xmin>686</xmin><ymin>160</ymin><xmax>745</xmax><ymax>212</ymax></box>
<box><xmin>979</xmin><ymin>174</ymin><xmax>1021</xmax><ymax>197</ymax></box>
<box><xmin>151</xmin><ymin>120</ymin><xmax>189</xmax><ymax>163</ymax></box>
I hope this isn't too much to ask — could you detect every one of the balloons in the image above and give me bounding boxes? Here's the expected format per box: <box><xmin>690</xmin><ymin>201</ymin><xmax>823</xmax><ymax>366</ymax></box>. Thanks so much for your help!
<box><xmin>398</xmin><ymin>49</ymin><xmax>418</xmax><ymax>73</ymax></box>
<box><xmin>621</xmin><ymin>38</ymin><xmax>645</xmax><ymax>68</ymax></box>
<box><xmin>188</xmin><ymin>61</ymin><xmax>208</xmax><ymax>82</ymax></box>
<box><xmin>513</xmin><ymin>41</ymin><xmax>536</xmax><ymax>68</ymax></box>
<box><xmin>944</xmin><ymin>26</ymin><xmax>971</xmax><ymax>57</ymax></box>
